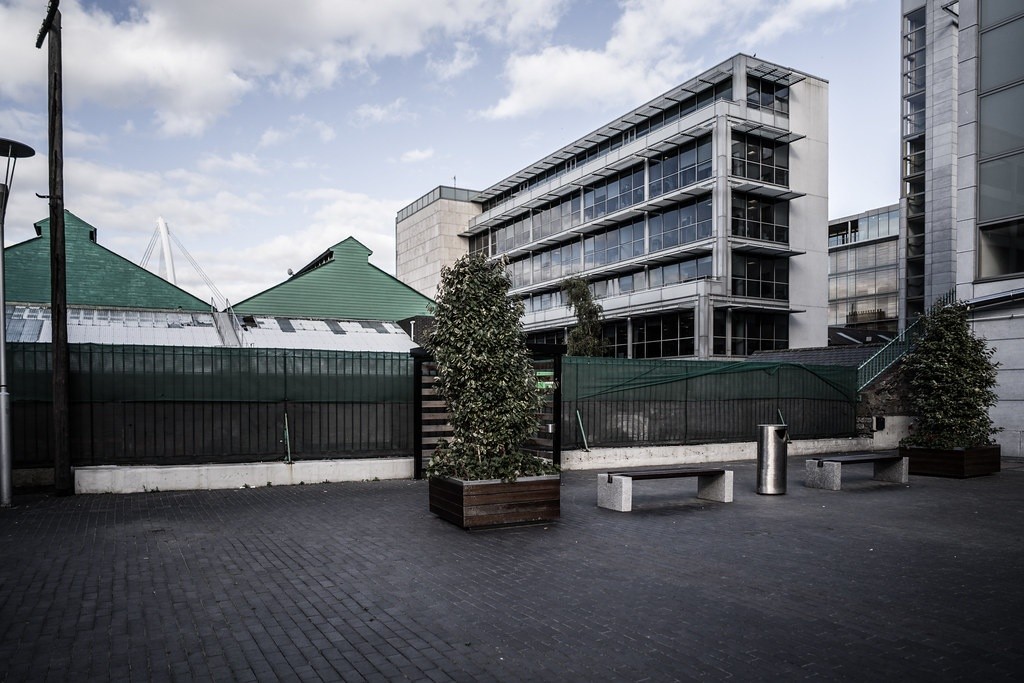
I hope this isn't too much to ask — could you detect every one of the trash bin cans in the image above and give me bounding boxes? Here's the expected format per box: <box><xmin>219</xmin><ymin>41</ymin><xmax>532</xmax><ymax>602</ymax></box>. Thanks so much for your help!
<box><xmin>755</xmin><ymin>424</ymin><xmax>789</xmax><ymax>495</ymax></box>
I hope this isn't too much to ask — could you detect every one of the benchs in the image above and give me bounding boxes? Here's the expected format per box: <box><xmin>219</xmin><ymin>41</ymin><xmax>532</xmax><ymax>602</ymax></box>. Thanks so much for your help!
<box><xmin>597</xmin><ymin>465</ymin><xmax>733</xmax><ymax>513</ymax></box>
<box><xmin>808</xmin><ymin>453</ymin><xmax>909</xmax><ymax>490</ymax></box>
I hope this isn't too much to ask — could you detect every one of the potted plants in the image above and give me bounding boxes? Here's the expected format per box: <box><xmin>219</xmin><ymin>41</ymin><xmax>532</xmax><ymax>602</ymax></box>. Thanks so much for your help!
<box><xmin>418</xmin><ymin>250</ymin><xmax>563</xmax><ymax>532</ymax></box>
<box><xmin>886</xmin><ymin>298</ymin><xmax>1005</xmax><ymax>480</ymax></box>
